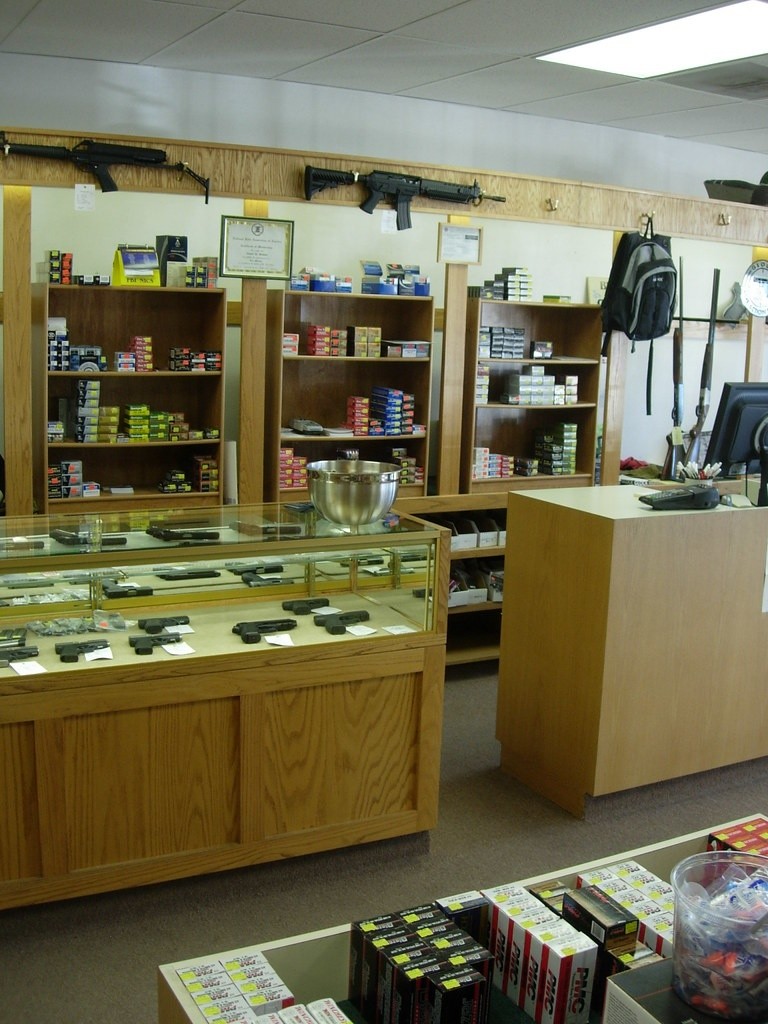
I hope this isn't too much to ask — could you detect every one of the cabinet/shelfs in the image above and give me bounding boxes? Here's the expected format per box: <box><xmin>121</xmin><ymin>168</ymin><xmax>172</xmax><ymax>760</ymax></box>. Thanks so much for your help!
<box><xmin>458</xmin><ymin>296</ymin><xmax>602</xmax><ymax>494</ymax></box>
<box><xmin>45</xmin><ymin>283</ymin><xmax>228</xmax><ymax>512</ymax></box>
<box><xmin>263</xmin><ymin>289</ymin><xmax>435</xmax><ymax>501</ymax></box>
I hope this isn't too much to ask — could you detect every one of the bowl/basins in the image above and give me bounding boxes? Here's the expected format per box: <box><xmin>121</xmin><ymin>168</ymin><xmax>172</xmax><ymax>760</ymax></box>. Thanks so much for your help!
<box><xmin>306</xmin><ymin>460</ymin><xmax>402</xmax><ymax>526</ymax></box>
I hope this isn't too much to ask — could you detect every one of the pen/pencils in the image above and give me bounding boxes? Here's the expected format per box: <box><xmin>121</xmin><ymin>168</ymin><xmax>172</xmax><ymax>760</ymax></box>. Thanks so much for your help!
<box><xmin>677</xmin><ymin>461</ymin><xmax>722</xmax><ymax>480</ymax></box>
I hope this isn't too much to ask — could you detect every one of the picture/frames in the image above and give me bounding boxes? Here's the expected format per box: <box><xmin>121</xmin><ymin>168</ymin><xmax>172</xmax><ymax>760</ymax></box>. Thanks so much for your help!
<box><xmin>437</xmin><ymin>222</ymin><xmax>483</xmax><ymax>265</ymax></box>
<box><xmin>219</xmin><ymin>215</ymin><xmax>294</xmax><ymax>281</ymax></box>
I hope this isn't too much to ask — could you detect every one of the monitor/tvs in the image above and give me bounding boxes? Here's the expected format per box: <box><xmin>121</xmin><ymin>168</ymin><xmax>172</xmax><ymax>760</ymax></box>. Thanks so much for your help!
<box><xmin>704</xmin><ymin>381</ymin><xmax>768</xmax><ymax>507</ymax></box>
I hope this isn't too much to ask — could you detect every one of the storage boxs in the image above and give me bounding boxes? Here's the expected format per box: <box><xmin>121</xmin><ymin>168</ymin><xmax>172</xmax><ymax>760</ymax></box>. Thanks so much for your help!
<box><xmin>472</xmin><ymin>423</ymin><xmax>578</xmax><ymax>480</ymax></box>
<box><xmin>114</xmin><ymin>336</ymin><xmax>154</xmax><ymax>371</ymax></box>
<box><xmin>179</xmin><ymin>817</ymin><xmax>768</xmax><ymax>1024</ymax></box>
<box><xmin>478</xmin><ymin>325</ymin><xmax>525</xmax><ymax>359</ymax></box>
<box><xmin>282</xmin><ymin>323</ymin><xmax>430</xmax><ymax>357</ymax></box>
<box><xmin>529</xmin><ymin>341</ymin><xmax>553</xmax><ymax>360</ymax></box>
<box><xmin>476</xmin><ymin>365</ymin><xmax>578</xmax><ymax>405</ymax></box>
<box><xmin>360</xmin><ymin>260</ymin><xmax>430</xmax><ymax>295</ymax></box>
<box><xmin>48</xmin><ymin>380</ymin><xmax>220</xmax><ymax>444</ymax></box>
<box><xmin>36</xmin><ymin>250</ymin><xmax>110</xmax><ymax>285</ymax></box>
<box><xmin>279</xmin><ymin>447</ymin><xmax>308</xmax><ymax>487</ymax></box>
<box><xmin>48</xmin><ymin>317</ymin><xmax>107</xmax><ymax>371</ymax></box>
<box><xmin>156</xmin><ymin>236</ymin><xmax>218</xmax><ymax>288</ymax></box>
<box><xmin>48</xmin><ymin>460</ymin><xmax>100</xmax><ymax>499</ymax></box>
<box><xmin>285</xmin><ymin>267</ymin><xmax>352</xmax><ymax>292</ymax></box>
<box><xmin>156</xmin><ymin>456</ymin><xmax>219</xmax><ymax>493</ymax></box>
<box><xmin>169</xmin><ymin>347</ymin><xmax>221</xmax><ymax>371</ymax></box>
<box><xmin>382</xmin><ymin>447</ymin><xmax>424</xmax><ymax>484</ymax></box>
<box><xmin>346</xmin><ymin>386</ymin><xmax>427</xmax><ymax>436</ymax></box>
<box><xmin>468</xmin><ymin>267</ymin><xmax>571</xmax><ymax>303</ymax></box>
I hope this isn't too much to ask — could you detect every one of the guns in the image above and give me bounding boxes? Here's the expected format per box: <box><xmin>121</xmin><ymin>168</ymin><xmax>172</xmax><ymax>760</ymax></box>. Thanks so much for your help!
<box><xmin>661</xmin><ymin>255</ymin><xmax>685</xmax><ymax>482</ymax></box>
<box><xmin>681</xmin><ymin>267</ymin><xmax>720</xmax><ymax>469</ymax></box>
<box><xmin>304</xmin><ymin>163</ymin><xmax>507</xmax><ymax>232</ymax></box>
<box><xmin>0</xmin><ymin>129</ymin><xmax>210</xmax><ymax>206</ymax></box>
<box><xmin>0</xmin><ymin>518</ymin><xmax>372</xmax><ymax>670</ymax></box>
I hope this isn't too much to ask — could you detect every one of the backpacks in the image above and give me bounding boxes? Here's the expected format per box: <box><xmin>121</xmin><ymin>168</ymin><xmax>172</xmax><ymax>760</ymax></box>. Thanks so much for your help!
<box><xmin>600</xmin><ymin>233</ymin><xmax>677</xmax><ymax>340</ymax></box>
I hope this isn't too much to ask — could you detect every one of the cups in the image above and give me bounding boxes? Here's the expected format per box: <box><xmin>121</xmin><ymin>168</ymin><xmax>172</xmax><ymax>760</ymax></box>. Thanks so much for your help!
<box><xmin>685</xmin><ymin>477</ymin><xmax>713</xmax><ymax>490</ymax></box>
<box><xmin>671</xmin><ymin>851</ymin><xmax>768</xmax><ymax>1017</ymax></box>
